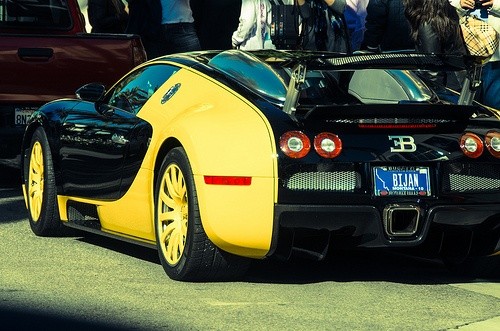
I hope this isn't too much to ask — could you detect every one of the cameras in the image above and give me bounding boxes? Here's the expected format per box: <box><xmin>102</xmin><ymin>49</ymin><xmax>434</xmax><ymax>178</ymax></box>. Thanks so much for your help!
<box><xmin>473</xmin><ymin>0</ymin><xmax>490</xmax><ymax>9</ymax></box>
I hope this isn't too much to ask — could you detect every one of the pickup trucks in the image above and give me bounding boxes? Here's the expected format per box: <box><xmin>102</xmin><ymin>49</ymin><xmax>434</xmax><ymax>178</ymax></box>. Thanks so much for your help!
<box><xmin>0</xmin><ymin>0</ymin><xmax>149</xmax><ymax>150</ymax></box>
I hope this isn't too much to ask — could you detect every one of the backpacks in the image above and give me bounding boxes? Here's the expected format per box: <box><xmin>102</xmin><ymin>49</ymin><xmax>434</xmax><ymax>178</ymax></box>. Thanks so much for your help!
<box><xmin>457</xmin><ymin>8</ymin><xmax>498</xmax><ymax>68</ymax></box>
<box><xmin>268</xmin><ymin>0</ymin><xmax>307</xmax><ymax>50</ymax></box>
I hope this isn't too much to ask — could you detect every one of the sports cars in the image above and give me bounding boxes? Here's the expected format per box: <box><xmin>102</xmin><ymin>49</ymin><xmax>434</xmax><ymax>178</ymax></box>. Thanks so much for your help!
<box><xmin>16</xmin><ymin>47</ymin><xmax>500</xmax><ymax>282</ymax></box>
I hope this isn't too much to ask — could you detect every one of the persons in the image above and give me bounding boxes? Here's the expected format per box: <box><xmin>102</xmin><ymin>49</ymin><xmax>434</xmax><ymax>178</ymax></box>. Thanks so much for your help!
<box><xmin>87</xmin><ymin>0</ymin><xmax>500</xmax><ymax>111</ymax></box>
